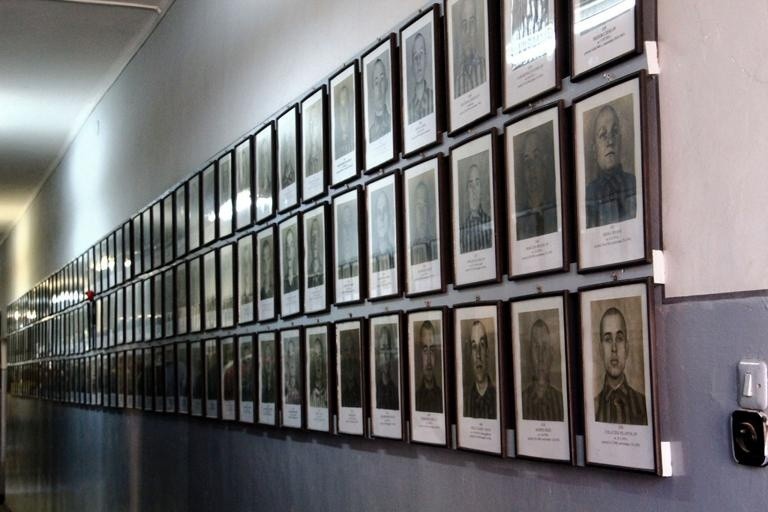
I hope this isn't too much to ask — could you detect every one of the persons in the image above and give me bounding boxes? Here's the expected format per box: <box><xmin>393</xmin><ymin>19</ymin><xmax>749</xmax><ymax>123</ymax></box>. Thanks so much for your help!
<box><xmin>515</xmin><ymin>131</ymin><xmax>558</xmax><ymax>240</ymax></box>
<box><xmin>454</xmin><ymin>0</ymin><xmax>486</xmax><ymax>97</ymax></box>
<box><xmin>594</xmin><ymin>307</ymin><xmax>649</xmax><ymax>426</ymax></box>
<box><xmin>408</xmin><ymin>32</ymin><xmax>433</xmax><ymax>124</ymax></box>
<box><xmin>585</xmin><ymin>104</ymin><xmax>637</xmax><ymax>228</ymax></box>
<box><xmin>464</xmin><ymin>320</ymin><xmax>498</xmax><ymax>419</ymax></box>
<box><xmin>414</xmin><ymin>321</ymin><xmax>444</xmax><ymax>413</ymax></box>
<box><xmin>410</xmin><ymin>182</ymin><xmax>438</xmax><ymax>263</ymax></box>
<box><xmin>522</xmin><ymin>318</ymin><xmax>564</xmax><ymax>421</ymax></box>
<box><xmin>458</xmin><ymin>163</ymin><xmax>493</xmax><ymax>255</ymax></box>
